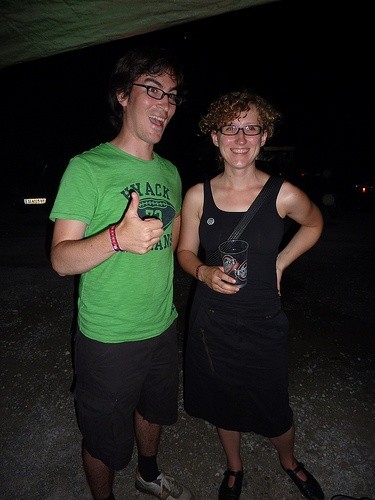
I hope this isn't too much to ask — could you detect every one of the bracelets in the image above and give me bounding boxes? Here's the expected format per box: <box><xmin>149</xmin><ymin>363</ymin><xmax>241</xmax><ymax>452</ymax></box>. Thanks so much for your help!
<box><xmin>109</xmin><ymin>224</ymin><xmax>121</xmax><ymax>252</ymax></box>
<box><xmin>195</xmin><ymin>264</ymin><xmax>207</xmax><ymax>283</ymax></box>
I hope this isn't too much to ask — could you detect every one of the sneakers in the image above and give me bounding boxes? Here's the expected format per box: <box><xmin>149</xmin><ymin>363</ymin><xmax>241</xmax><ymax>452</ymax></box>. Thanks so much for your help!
<box><xmin>135</xmin><ymin>465</ymin><xmax>194</xmax><ymax>500</ymax></box>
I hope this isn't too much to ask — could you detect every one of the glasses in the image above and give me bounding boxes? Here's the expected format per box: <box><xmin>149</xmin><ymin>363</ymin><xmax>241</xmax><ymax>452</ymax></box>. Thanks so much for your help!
<box><xmin>216</xmin><ymin>125</ymin><xmax>265</xmax><ymax>136</ymax></box>
<box><xmin>131</xmin><ymin>83</ymin><xmax>182</xmax><ymax>105</ymax></box>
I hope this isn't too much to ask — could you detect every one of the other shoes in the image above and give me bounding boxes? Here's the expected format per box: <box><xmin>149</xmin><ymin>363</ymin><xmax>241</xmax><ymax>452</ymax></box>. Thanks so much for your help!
<box><xmin>282</xmin><ymin>458</ymin><xmax>325</xmax><ymax>500</ymax></box>
<box><xmin>218</xmin><ymin>467</ymin><xmax>244</xmax><ymax>500</ymax></box>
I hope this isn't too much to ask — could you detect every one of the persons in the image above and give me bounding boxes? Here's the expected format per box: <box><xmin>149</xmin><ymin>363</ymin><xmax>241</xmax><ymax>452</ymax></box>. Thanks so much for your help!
<box><xmin>49</xmin><ymin>52</ymin><xmax>192</xmax><ymax>500</ymax></box>
<box><xmin>177</xmin><ymin>91</ymin><xmax>324</xmax><ymax>500</ymax></box>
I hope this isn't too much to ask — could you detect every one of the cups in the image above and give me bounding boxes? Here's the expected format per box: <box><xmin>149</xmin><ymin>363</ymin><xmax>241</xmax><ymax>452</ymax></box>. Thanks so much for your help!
<box><xmin>219</xmin><ymin>240</ymin><xmax>249</xmax><ymax>288</ymax></box>
